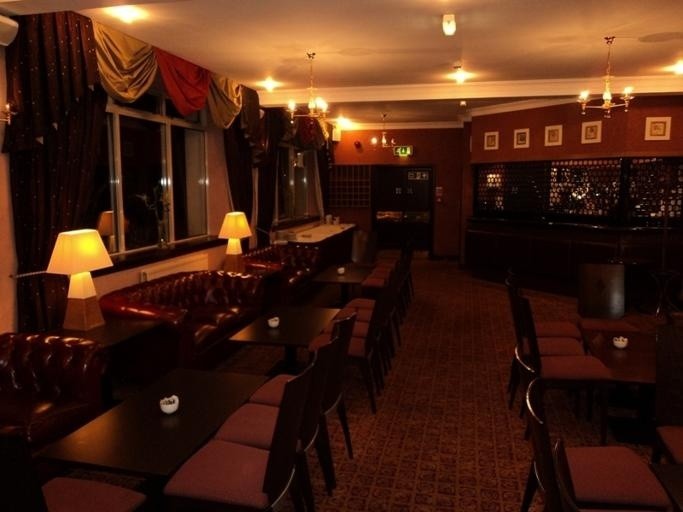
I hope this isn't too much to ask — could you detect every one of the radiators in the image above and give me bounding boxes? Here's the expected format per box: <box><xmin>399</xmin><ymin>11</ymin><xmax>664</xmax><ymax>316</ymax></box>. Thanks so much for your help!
<box><xmin>140</xmin><ymin>254</ymin><xmax>211</xmax><ymax>281</ymax></box>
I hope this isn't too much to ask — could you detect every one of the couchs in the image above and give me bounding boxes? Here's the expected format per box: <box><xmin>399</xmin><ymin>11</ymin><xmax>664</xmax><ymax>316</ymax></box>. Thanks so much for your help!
<box><xmin>242</xmin><ymin>246</ymin><xmax>323</xmax><ymax>294</ymax></box>
<box><xmin>0</xmin><ymin>334</ymin><xmax>98</xmax><ymax>461</ymax></box>
<box><xmin>99</xmin><ymin>272</ymin><xmax>257</xmax><ymax>351</ymax></box>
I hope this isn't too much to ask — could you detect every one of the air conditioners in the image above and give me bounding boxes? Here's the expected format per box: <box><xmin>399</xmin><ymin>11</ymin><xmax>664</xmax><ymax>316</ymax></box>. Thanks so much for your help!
<box><xmin>0</xmin><ymin>15</ymin><xmax>19</xmax><ymax>47</ymax></box>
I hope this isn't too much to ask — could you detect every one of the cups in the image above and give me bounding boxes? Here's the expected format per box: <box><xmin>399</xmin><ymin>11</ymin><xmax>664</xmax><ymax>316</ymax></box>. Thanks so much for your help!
<box><xmin>338</xmin><ymin>267</ymin><xmax>344</xmax><ymax>274</ymax></box>
<box><xmin>613</xmin><ymin>336</ymin><xmax>628</xmax><ymax>349</ymax></box>
<box><xmin>268</xmin><ymin>317</ymin><xmax>279</xmax><ymax>327</ymax></box>
<box><xmin>160</xmin><ymin>395</ymin><xmax>179</xmax><ymax>414</ymax></box>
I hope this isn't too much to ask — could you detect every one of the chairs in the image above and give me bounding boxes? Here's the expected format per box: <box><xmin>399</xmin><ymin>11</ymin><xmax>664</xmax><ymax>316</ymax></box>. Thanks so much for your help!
<box><xmin>310</xmin><ymin>285</ymin><xmax>399</xmax><ymax>414</ymax></box>
<box><xmin>0</xmin><ymin>476</ymin><xmax>140</xmax><ymax>511</ymax></box>
<box><xmin>522</xmin><ymin>379</ymin><xmax>654</xmax><ymax>512</ymax></box>
<box><xmin>508</xmin><ymin>279</ymin><xmax>616</xmax><ymax>441</ymax></box>
<box><xmin>162</xmin><ymin>313</ymin><xmax>359</xmax><ymax>511</ymax></box>
<box><xmin>364</xmin><ymin>241</ymin><xmax>415</xmax><ymax>323</ymax></box>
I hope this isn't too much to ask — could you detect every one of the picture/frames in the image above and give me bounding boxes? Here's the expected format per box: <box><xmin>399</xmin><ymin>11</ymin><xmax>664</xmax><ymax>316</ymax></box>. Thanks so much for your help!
<box><xmin>0</xmin><ymin>98</ymin><xmax>19</xmax><ymax>125</ymax></box>
<box><xmin>644</xmin><ymin>117</ymin><xmax>671</xmax><ymax>140</ymax></box>
<box><xmin>545</xmin><ymin>125</ymin><xmax>562</xmax><ymax>146</ymax></box>
<box><xmin>582</xmin><ymin>121</ymin><xmax>602</xmax><ymax>144</ymax></box>
<box><xmin>513</xmin><ymin>128</ymin><xmax>530</xmax><ymax>149</ymax></box>
<box><xmin>484</xmin><ymin>132</ymin><xmax>499</xmax><ymax>150</ymax></box>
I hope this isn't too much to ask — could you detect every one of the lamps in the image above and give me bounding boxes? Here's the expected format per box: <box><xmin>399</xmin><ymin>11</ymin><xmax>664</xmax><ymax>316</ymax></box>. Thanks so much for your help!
<box><xmin>46</xmin><ymin>228</ymin><xmax>114</xmax><ymax>331</ymax></box>
<box><xmin>97</xmin><ymin>211</ymin><xmax>129</xmax><ymax>254</ymax></box>
<box><xmin>441</xmin><ymin>13</ymin><xmax>458</xmax><ymax>36</ymax></box>
<box><xmin>286</xmin><ymin>49</ymin><xmax>331</xmax><ymax>127</ymax></box>
<box><xmin>369</xmin><ymin>112</ymin><xmax>396</xmax><ymax>152</ymax></box>
<box><xmin>218</xmin><ymin>211</ymin><xmax>254</xmax><ymax>274</ymax></box>
<box><xmin>576</xmin><ymin>36</ymin><xmax>634</xmax><ymax>118</ymax></box>
